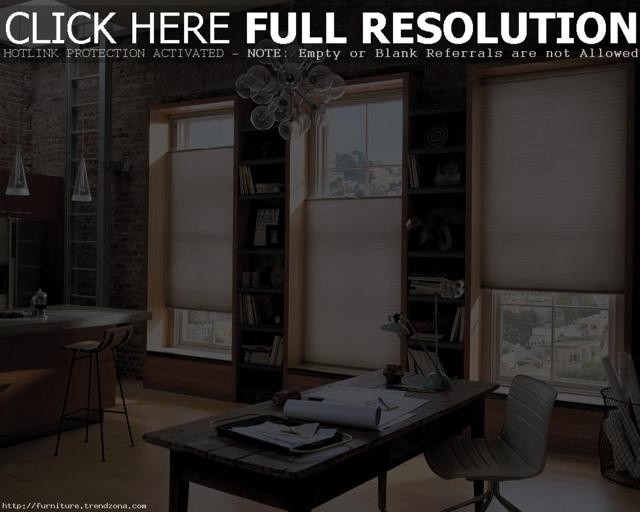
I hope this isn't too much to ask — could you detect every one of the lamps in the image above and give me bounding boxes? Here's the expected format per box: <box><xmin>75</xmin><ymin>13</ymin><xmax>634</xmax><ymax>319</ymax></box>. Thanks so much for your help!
<box><xmin>5</xmin><ymin>0</ymin><xmax>30</xmax><ymax>196</ymax></box>
<box><xmin>233</xmin><ymin>40</ymin><xmax>345</xmax><ymax>142</ymax></box>
<box><xmin>71</xmin><ymin>0</ymin><xmax>93</xmax><ymax>201</ymax></box>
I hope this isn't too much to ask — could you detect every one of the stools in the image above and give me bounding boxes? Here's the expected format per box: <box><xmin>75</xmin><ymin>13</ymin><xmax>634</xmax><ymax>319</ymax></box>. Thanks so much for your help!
<box><xmin>54</xmin><ymin>325</ymin><xmax>135</xmax><ymax>462</ymax></box>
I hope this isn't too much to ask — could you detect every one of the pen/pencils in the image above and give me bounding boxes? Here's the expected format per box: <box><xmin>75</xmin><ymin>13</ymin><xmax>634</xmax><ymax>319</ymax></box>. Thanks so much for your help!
<box><xmin>387</xmin><ymin>384</ymin><xmax>426</xmax><ymax>390</ymax></box>
<box><xmin>378</xmin><ymin>397</ymin><xmax>388</xmax><ymax>408</ymax></box>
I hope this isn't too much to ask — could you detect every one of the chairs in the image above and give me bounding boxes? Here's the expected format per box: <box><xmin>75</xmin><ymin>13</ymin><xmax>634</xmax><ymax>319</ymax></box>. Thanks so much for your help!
<box><xmin>423</xmin><ymin>374</ymin><xmax>557</xmax><ymax>511</ymax></box>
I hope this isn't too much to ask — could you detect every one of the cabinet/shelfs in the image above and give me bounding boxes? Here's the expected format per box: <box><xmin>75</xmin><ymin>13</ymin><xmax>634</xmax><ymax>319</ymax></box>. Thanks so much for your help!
<box><xmin>400</xmin><ymin>71</ymin><xmax>473</xmax><ymax>379</ymax></box>
<box><xmin>232</xmin><ymin>96</ymin><xmax>290</xmax><ymax>404</ymax></box>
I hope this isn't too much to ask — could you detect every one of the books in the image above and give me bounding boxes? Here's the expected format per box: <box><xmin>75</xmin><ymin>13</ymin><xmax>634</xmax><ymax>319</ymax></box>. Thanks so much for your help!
<box><xmin>407</xmin><ymin>152</ymin><xmax>419</xmax><ymax>189</ymax></box>
<box><xmin>238</xmin><ymin>165</ymin><xmax>282</xmax><ymax>195</ymax></box>
<box><xmin>239</xmin><ymin>293</ymin><xmax>285</xmax><ymax>366</ymax></box>
<box><xmin>406</xmin><ymin>274</ymin><xmax>465</xmax><ymax>378</ymax></box>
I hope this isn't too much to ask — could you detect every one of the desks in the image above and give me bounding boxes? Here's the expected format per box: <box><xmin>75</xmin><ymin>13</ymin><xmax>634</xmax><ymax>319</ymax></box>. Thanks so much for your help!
<box><xmin>141</xmin><ymin>369</ymin><xmax>499</xmax><ymax>512</ymax></box>
<box><xmin>0</xmin><ymin>304</ymin><xmax>152</xmax><ymax>439</ymax></box>
